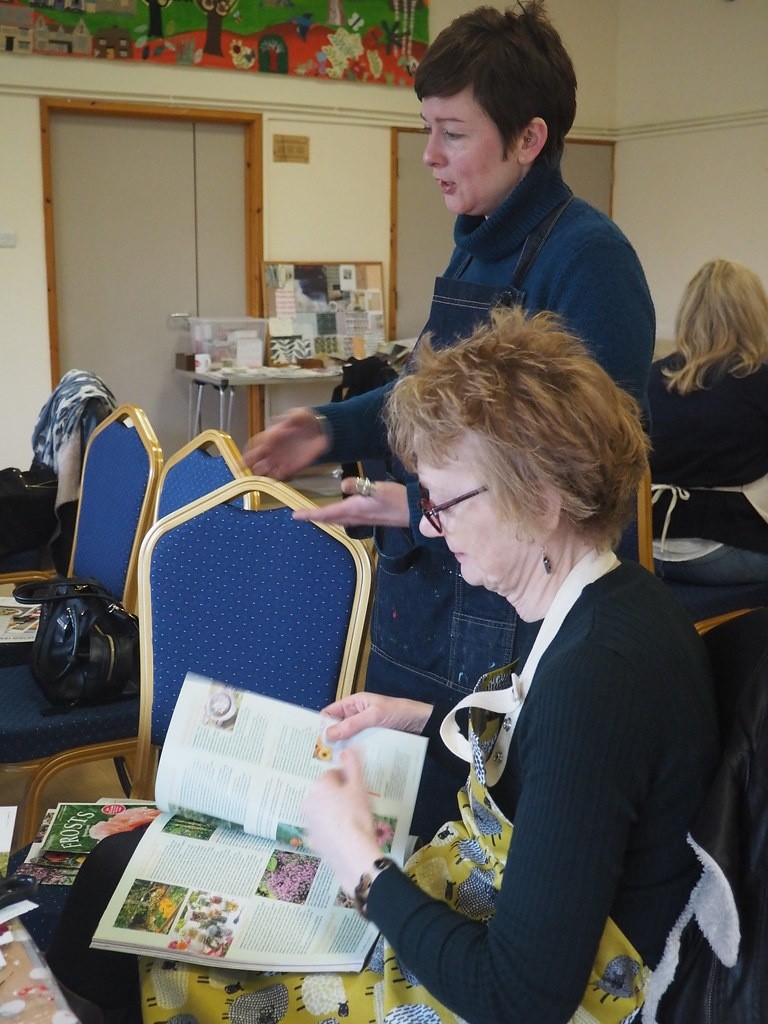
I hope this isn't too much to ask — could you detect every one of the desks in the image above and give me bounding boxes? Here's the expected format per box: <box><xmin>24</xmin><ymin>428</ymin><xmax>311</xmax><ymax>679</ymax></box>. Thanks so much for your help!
<box><xmin>171</xmin><ymin>368</ymin><xmax>343</xmax><ymax>444</ymax></box>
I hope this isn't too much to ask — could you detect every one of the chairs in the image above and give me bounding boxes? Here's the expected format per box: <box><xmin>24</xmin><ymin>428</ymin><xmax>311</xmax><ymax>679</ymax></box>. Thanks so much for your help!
<box><xmin>0</xmin><ymin>403</ymin><xmax>164</xmax><ymax>668</ymax></box>
<box><xmin>612</xmin><ymin>462</ymin><xmax>768</xmax><ymax>638</ymax></box>
<box><xmin>128</xmin><ymin>476</ymin><xmax>377</xmax><ymax>798</ymax></box>
<box><xmin>0</xmin><ymin>368</ymin><xmax>119</xmax><ymax>594</ymax></box>
<box><xmin>0</xmin><ymin>428</ymin><xmax>261</xmax><ymax>850</ymax></box>
<box><xmin>640</xmin><ymin>606</ymin><xmax>768</xmax><ymax>1024</ymax></box>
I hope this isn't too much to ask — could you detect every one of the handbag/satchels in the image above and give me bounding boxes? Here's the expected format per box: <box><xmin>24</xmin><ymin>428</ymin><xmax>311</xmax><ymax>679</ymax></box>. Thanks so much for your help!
<box><xmin>12</xmin><ymin>579</ymin><xmax>139</xmax><ymax>717</ymax></box>
<box><xmin>0</xmin><ymin>463</ymin><xmax>58</xmax><ymax>559</ymax></box>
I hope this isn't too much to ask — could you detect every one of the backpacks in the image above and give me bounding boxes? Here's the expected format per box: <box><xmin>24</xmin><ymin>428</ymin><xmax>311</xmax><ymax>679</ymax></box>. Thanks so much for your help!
<box><xmin>330</xmin><ymin>356</ymin><xmax>400</xmax><ymax>477</ymax></box>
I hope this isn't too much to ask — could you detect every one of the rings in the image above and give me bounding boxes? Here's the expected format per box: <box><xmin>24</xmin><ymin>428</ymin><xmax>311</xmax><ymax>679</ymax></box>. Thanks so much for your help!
<box><xmin>357</xmin><ymin>477</ymin><xmax>377</xmax><ymax>496</ymax></box>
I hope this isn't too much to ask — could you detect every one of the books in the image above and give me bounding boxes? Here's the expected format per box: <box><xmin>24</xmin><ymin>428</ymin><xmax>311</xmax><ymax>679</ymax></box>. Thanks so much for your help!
<box><xmin>87</xmin><ymin>668</ymin><xmax>429</xmax><ymax>977</ymax></box>
<box><xmin>0</xmin><ymin>794</ymin><xmax>159</xmax><ymax>886</ymax></box>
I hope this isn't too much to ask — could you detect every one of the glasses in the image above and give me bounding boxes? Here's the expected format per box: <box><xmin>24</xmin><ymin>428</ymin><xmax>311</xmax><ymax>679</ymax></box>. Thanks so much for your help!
<box><xmin>417</xmin><ymin>483</ymin><xmax>489</xmax><ymax>534</ymax></box>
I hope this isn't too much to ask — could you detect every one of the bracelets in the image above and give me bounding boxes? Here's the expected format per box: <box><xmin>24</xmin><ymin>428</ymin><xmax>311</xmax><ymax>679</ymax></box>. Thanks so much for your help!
<box><xmin>354</xmin><ymin>857</ymin><xmax>394</xmax><ymax>917</ymax></box>
<box><xmin>312</xmin><ymin>410</ymin><xmax>333</xmax><ymax>455</ymax></box>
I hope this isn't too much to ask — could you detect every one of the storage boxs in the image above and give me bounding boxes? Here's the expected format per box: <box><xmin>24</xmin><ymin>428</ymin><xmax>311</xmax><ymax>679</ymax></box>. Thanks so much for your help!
<box><xmin>187</xmin><ymin>316</ymin><xmax>269</xmax><ymax>372</ymax></box>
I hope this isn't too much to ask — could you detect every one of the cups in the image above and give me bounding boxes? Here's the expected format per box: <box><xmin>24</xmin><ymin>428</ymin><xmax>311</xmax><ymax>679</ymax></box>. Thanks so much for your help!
<box><xmin>194</xmin><ymin>354</ymin><xmax>212</xmax><ymax>373</ymax></box>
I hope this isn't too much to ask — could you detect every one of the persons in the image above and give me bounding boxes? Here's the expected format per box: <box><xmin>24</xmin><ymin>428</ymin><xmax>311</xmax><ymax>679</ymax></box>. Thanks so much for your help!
<box><xmin>232</xmin><ymin>3</ymin><xmax>657</xmax><ymax>700</ymax></box>
<box><xmin>56</xmin><ymin>308</ymin><xmax>718</xmax><ymax>1023</ymax></box>
<box><xmin>648</xmin><ymin>257</ymin><xmax>767</xmax><ymax>556</ymax></box>
